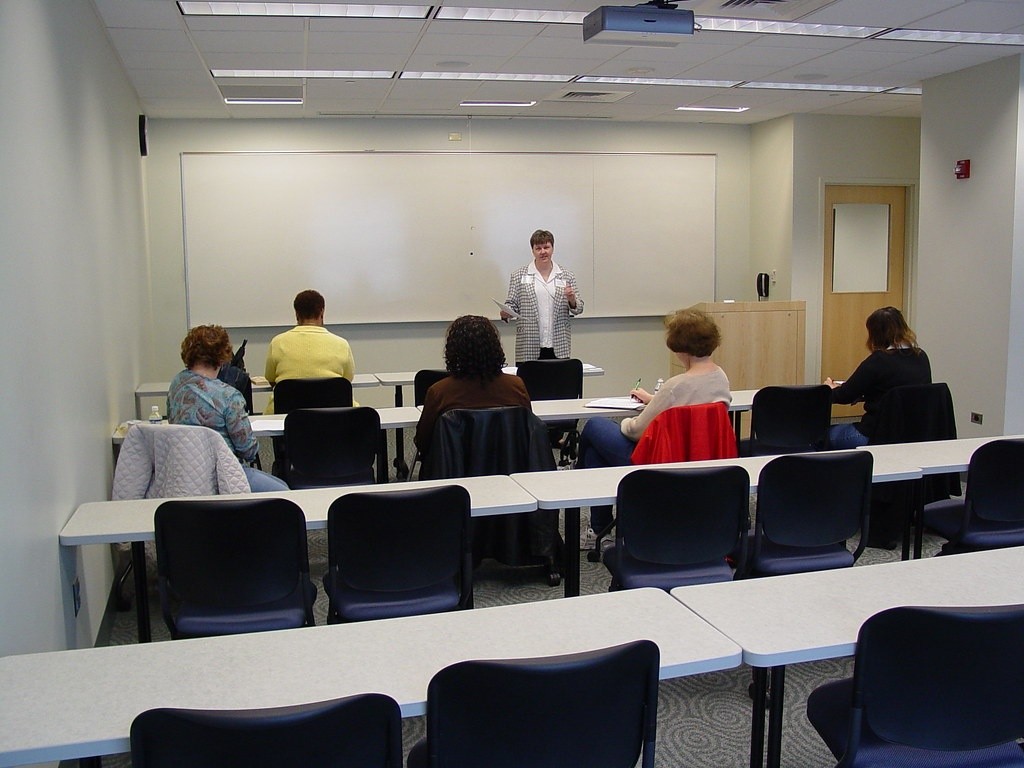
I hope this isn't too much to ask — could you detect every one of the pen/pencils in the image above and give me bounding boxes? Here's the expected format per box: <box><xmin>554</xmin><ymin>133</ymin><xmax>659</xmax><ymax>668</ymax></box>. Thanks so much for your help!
<box><xmin>630</xmin><ymin>378</ymin><xmax>642</xmax><ymax>399</ymax></box>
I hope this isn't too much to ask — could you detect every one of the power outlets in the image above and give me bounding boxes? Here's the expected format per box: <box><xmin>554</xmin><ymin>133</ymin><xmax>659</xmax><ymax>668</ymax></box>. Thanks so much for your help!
<box><xmin>971</xmin><ymin>411</ymin><xmax>983</xmax><ymax>426</ymax></box>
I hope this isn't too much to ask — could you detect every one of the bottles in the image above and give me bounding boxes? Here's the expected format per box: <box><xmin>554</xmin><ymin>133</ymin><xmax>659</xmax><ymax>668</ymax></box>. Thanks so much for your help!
<box><xmin>149</xmin><ymin>406</ymin><xmax>162</xmax><ymax>424</ymax></box>
<box><xmin>655</xmin><ymin>379</ymin><xmax>664</xmax><ymax>394</ymax></box>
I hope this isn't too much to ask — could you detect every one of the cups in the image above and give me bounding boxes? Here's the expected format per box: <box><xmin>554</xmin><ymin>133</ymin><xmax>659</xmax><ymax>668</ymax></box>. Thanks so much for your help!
<box><xmin>757</xmin><ymin>272</ymin><xmax>769</xmax><ymax>301</ymax></box>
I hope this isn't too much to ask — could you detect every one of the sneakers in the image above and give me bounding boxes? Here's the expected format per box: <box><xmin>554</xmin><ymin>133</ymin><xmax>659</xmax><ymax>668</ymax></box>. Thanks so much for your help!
<box><xmin>562</xmin><ymin>528</ymin><xmax>616</xmax><ymax>551</ymax></box>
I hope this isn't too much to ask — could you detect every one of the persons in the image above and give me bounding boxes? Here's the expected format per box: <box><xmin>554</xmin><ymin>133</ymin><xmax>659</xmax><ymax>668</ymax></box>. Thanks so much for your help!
<box><xmin>166</xmin><ymin>325</ymin><xmax>291</xmax><ymax>493</ymax></box>
<box><xmin>500</xmin><ymin>229</ymin><xmax>584</xmax><ymax>449</ymax></box>
<box><xmin>261</xmin><ymin>290</ymin><xmax>361</xmax><ymax>475</ymax></box>
<box><xmin>413</xmin><ymin>315</ymin><xmax>532</xmax><ymax>464</ymax></box>
<box><xmin>557</xmin><ymin>308</ymin><xmax>733</xmax><ymax>552</ymax></box>
<box><xmin>822</xmin><ymin>306</ymin><xmax>932</xmax><ymax>450</ymax></box>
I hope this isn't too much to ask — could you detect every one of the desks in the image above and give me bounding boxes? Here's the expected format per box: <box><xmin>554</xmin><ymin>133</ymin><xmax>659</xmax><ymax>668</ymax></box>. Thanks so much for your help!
<box><xmin>0</xmin><ymin>361</ymin><xmax>1024</xmax><ymax>768</ymax></box>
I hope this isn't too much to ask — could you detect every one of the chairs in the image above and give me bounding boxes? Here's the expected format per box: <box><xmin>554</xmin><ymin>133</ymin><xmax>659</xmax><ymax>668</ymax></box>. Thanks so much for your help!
<box><xmin>587</xmin><ymin>401</ymin><xmax>738</xmax><ymax>562</ymax></box>
<box><xmin>323</xmin><ymin>484</ymin><xmax>473</xmax><ymax>625</ymax></box>
<box><xmin>274</xmin><ymin>377</ymin><xmax>352</xmax><ymax>414</ymax></box>
<box><xmin>924</xmin><ymin>438</ymin><xmax>1024</xmax><ymax>557</ymax></box>
<box><xmin>418</xmin><ymin>407</ymin><xmax>572</xmax><ymax>587</ymax></box>
<box><xmin>154</xmin><ymin>495</ymin><xmax>318</xmax><ymax>641</ymax></box>
<box><xmin>866</xmin><ymin>382</ymin><xmax>958</xmax><ymax>561</ymax></box>
<box><xmin>804</xmin><ymin>604</ymin><xmax>1024</xmax><ymax>768</ymax></box>
<box><xmin>748</xmin><ymin>451</ymin><xmax>875</xmax><ymax>581</ymax></box>
<box><xmin>273</xmin><ymin>408</ymin><xmax>391</xmax><ymax>497</ymax></box>
<box><xmin>601</xmin><ymin>468</ymin><xmax>752</xmax><ymax>599</ymax></box>
<box><xmin>407</xmin><ymin>370</ymin><xmax>449</xmax><ymax>481</ymax></box>
<box><xmin>407</xmin><ymin>641</ymin><xmax>659</xmax><ymax>768</ymax></box>
<box><xmin>129</xmin><ymin>691</ymin><xmax>402</xmax><ymax>768</ymax></box>
<box><xmin>746</xmin><ymin>385</ymin><xmax>835</xmax><ymax>457</ymax></box>
<box><xmin>516</xmin><ymin>358</ymin><xmax>585</xmax><ymax>466</ymax></box>
<box><xmin>217</xmin><ymin>370</ymin><xmax>262</xmax><ymax>472</ymax></box>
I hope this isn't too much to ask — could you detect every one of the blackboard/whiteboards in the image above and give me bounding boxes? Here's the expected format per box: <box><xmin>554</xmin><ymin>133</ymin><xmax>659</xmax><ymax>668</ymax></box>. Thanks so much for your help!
<box><xmin>180</xmin><ymin>149</ymin><xmax>717</xmax><ymax>331</ymax></box>
